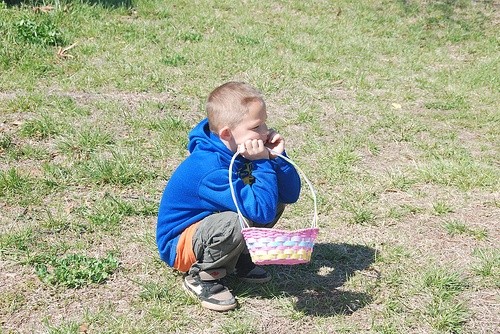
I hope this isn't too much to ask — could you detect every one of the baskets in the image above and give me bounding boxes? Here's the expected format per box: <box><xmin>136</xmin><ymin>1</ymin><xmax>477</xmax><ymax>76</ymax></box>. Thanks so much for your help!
<box><xmin>229</xmin><ymin>147</ymin><xmax>319</xmax><ymax>266</ymax></box>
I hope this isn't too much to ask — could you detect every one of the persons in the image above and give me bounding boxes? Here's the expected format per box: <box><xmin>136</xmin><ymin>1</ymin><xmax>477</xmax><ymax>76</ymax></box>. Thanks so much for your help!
<box><xmin>155</xmin><ymin>80</ymin><xmax>303</xmax><ymax>312</ymax></box>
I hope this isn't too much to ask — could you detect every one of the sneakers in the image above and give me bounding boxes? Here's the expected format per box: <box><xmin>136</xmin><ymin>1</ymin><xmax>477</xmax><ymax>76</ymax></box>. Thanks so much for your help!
<box><xmin>229</xmin><ymin>266</ymin><xmax>272</xmax><ymax>284</ymax></box>
<box><xmin>181</xmin><ymin>274</ymin><xmax>237</xmax><ymax>311</ymax></box>
